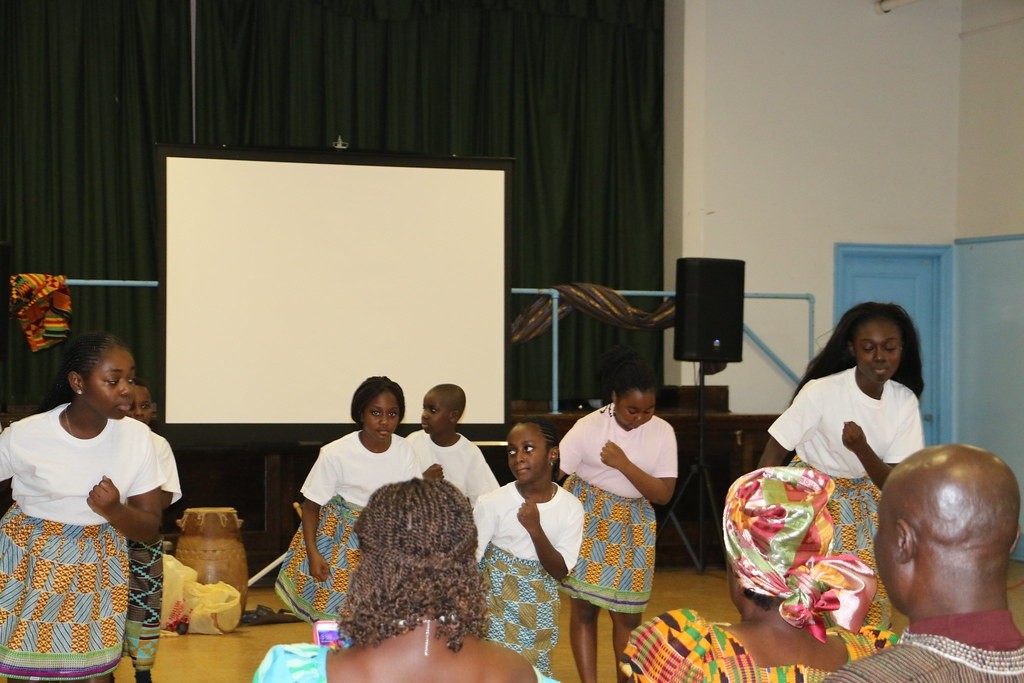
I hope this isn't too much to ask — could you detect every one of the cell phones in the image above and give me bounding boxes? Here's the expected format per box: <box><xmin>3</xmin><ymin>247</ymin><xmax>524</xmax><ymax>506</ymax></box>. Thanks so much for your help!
<box><xmin>312</xmin><ymin>620</ymin><xmax>350</xmax><ymax>649</ymax></box>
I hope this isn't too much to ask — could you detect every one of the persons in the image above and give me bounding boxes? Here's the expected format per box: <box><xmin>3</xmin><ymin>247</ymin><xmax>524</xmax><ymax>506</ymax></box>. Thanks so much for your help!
<box><xmin>619</xmin><ymin>467</ymin><xmax>901</xmax><ymax>683</ymax></box>
<box><xmin>100</xmin><ymin>380</ymin><xmax>182</xmax><ymax>683</ymax></box>
<box><xmin>272</xmin><ymin>375</ymin><xmax>426</xmax><ymax>635</ymax></box>
<box><xmin>469</xmin><ymin>421</ymin><xmax>585</xmax><ymax>683</ymax></box>
<box><xmin>825</xmin><ymin>442</ymin><xmax>1024</xmax><ymax>683</ymax></box>
<box><xmin>402</xmin><ymin>381</ymin><xmax>503</xmax><ymax>508</ymax></box>
<box><xmin>755</xmin><ymin>300</ymin><xmax>930</xmax><ymax>642</ymax></box>
<box><xmin>251</xmin><ymin>475</ymin><xmax>540</xmax><ymax>683</ymax></box>
<box><xmin>551</xmin><ymin>341</ymin><xmax>681</xmax><ymax>682</ymax></box>
<box><xmin>0</xmin><ymin>330</ymin><xmax>169</xmax><ymax>682</ymax></box>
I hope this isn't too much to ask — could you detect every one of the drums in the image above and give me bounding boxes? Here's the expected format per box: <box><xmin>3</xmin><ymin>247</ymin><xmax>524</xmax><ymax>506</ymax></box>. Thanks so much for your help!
<box><xmin>174</xmin><ymin>505</ymin><xmax>250</xmax><ymax>627</ymax></box>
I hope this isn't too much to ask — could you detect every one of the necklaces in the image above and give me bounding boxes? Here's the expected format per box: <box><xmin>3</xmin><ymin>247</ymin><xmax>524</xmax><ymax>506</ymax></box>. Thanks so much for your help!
<box><xmin>548</xmin><ymin>481</ymin><xmax>557</xmax><ymax>500</ymax></box>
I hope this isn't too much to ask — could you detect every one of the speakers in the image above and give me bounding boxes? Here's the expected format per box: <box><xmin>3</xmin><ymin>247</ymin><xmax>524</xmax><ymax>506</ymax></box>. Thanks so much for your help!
<box><xmin>674</xmin><ymin>257</ymin><xmax>745</xmax><ymax>363</ymax></box>
